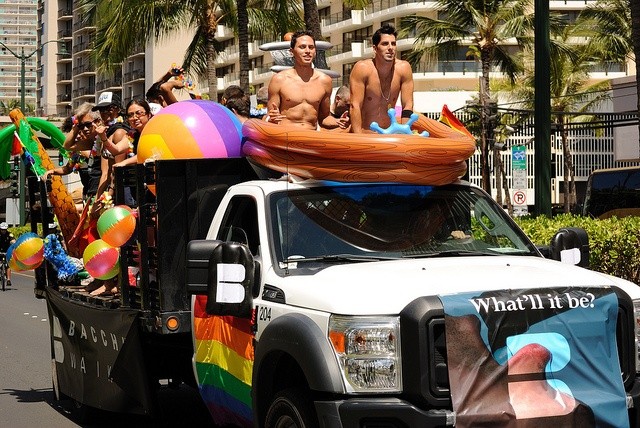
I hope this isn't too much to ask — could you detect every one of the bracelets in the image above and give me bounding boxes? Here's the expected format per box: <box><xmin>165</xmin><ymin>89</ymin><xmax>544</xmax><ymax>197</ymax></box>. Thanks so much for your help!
<box><xmin>101</xmin><ymin>138</ymin><xmax>108</xmax><ymax>142</ymax></box>
<box><xmin>109</xmin><ymin>185</ymin><xmax>114</xmax><ymax>190</ymax></box>
<box><xmin>53</xmin><ymin>169</ymin><xmax>56</xmax><ymax>175</ymax></box>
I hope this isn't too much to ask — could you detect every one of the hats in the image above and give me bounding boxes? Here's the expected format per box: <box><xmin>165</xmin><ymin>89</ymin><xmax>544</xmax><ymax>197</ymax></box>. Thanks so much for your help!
<box><xmin>91</xmin><ymin>91</ymin><xmax>121</xmax><ymax>112</ymax></box>
<box><xmin>0</xmin><ymin>222</ymin><xmax>8</xmax><ymax>230</ymax></box>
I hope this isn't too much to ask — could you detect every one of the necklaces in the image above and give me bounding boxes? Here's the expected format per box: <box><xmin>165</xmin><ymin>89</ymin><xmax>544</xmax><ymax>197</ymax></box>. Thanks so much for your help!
<box><xmin>371</xmin><ymin>60</ymin><xmax>395</xmax><ymax>109</ymax></box>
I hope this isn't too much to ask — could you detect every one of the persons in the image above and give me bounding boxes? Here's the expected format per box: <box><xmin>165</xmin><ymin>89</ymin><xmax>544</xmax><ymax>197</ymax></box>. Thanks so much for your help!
<box><xmin>256</xmin><ymin>84</ymin><xmax>268</xmax><ymax>109</ymax></box>
<box><xmin>42</xmin><ymin>116</ymin><xmax>86</xmax><ymax>184</ymax></box>
<box><xmin>268</xmin><ymin>32</ymin><xmax>351</xmax><ymax>131</ymax></box>
<box><xmin>329</xmin><ymin>85</ymin><xmax>351</xmax><ymax>118</ymax></box>
<box><xmin>413</xmin><ymin>203</ymin><xmax>471</xmax><ymax>241</ymax></box>
<box><xmin>222</xmin><ymin>85</ymin><xmax>244</xmax><ymax>107</ymax></box>
<box><xmin>63</xmin><ymin>102</ymin><xmax>103</xmax><ymax>249</ymax></box>
<box><xmin>93</xmin><ymin>99</ymin><xmax>151</xmax><ymax>156</ymax></box>
<box><xmin>351</xmin><ymin>25</ymin><xmax>414</xmax><ymax>134</ymax></box>
<box><xmin>273</xmin><ymin>202</ymin><xmax>303</xmax><ymax>260</ymax></box>
<box><xmin>82</xmin><ymin>92</ymin><xmax>131</xmax><ymax>296</ymax></box>
<box><xmin>0</xmin><ymin>222</ymin><xmax>17</xmax><ymax>286</ymax></box>
<box><xmin>146</xmin><ymin>65</ymin><xmax>187</xmax><ymax>108</ymax></box>
<box><xmin>227</xmin><ymin>95</ymin><xmax>250</xmax><ymax>123</ymax></box>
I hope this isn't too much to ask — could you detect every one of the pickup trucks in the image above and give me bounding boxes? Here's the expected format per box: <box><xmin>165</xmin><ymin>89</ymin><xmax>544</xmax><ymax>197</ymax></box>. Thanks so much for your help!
<box><xmin>39</xmin><ymin>158</ymin><xmax>640</xmax><ymax>427</ymax></box>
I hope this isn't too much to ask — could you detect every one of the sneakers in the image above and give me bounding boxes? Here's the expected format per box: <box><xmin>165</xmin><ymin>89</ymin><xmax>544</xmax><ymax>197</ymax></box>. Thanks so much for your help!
<box><xmin>7</xmin><ymin>280</ymin><xmax>11</xmax><ymax>286</ymax></box>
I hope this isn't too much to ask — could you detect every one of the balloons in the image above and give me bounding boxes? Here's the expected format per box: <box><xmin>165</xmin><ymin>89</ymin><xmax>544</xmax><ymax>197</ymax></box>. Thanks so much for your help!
<box><xmin>138</xmin><ymin>100</ymin><xmax>244</xmax><ymax>162</ymax></box>
<box><xmin>97</xmin><ymin>205</ymin><xmax>136</xmax><ymax>247</ymax></box>
<box><xmin>95</xmin><ymin>261</ymin><xmax>121</xmax><ymax>281</ymax></box>
<box><xmin>6</xmin><ymin>243</ymin><xmax>30</xmax><ymax>272</ymax></box>
<box><xmin>83</xmin><ymin>239</ymin><xmax>119</xmax><ymax>278</ymax></box>
<box><xmin>15</xmin><ymin>232</ymin><xmax>46</xmax><ymax>264</ymax></box>
<box><xmin>12</xmin><ymin>249</ymin><xmax>44</xmax><ymax>270</ymax></box>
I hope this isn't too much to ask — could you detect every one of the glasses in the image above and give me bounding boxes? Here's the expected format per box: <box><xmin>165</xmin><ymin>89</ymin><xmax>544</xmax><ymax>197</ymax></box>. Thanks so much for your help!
<box><xmin>77</xmin><ymin>118</ymin><xmax>97</xmax><ymax>130</ymax></box>
<box><xmin>127</xmin><ymin>111</ymin><xmax>147</xmax><ymax>118</ymax></box>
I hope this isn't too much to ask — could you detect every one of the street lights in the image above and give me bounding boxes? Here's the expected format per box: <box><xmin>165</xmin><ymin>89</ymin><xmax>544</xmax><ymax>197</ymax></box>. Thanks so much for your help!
<box><xmin>0</xmin><ymin>39</ymin><xmax>70</xmax><ymax>224</ymax></box>
